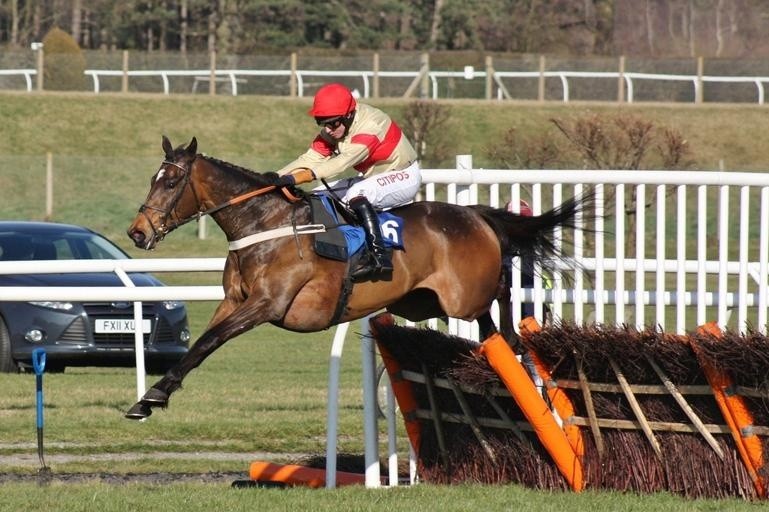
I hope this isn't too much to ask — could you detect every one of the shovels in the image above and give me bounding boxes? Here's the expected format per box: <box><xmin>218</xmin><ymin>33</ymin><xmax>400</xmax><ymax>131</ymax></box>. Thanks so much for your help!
<box><xmin>32</xmin><ymin>348</ymin><xmax>52</xmax><ymax>488</ymax></box>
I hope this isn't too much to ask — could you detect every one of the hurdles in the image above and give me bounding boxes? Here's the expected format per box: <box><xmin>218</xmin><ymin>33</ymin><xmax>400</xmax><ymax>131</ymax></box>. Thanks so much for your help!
<box><xmin>234</xmin><ymin>162</ymin><xmax>769</xmax><ymax>501</ymax></box>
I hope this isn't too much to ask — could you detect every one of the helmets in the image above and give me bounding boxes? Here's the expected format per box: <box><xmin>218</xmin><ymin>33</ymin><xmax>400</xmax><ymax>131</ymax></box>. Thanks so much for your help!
<box><xmin>307</xmin><ymin>82</ymin><xmax>356</xmax><ymax>117</ymax></box>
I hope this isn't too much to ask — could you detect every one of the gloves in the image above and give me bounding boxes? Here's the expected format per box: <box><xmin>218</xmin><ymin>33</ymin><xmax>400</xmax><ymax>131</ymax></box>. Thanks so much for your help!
<box><xmin>273</xmin><ymin>175</ymin><xmax>293</xmax><ymax>188</ymax></box>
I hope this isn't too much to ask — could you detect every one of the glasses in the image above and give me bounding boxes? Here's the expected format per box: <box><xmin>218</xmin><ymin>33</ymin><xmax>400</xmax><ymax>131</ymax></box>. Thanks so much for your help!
<box><xmin>315</xmin><ymin>118</ymin><xmax>343</xmax><ymax>129</ymax></box>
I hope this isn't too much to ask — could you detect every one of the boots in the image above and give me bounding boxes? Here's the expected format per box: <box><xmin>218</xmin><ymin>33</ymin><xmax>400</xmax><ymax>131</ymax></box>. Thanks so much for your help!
<box><xmin>351</xmin><ymin>198</ymin><xmax>393</xmax><ymax>277</ymax></box>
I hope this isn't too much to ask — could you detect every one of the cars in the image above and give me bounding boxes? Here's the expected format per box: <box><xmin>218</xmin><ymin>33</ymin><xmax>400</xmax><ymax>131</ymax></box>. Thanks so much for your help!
<box><xmin>0</xmin><ymin>220</ymin><xmax>189</xmax><ymax>374</ymax></box>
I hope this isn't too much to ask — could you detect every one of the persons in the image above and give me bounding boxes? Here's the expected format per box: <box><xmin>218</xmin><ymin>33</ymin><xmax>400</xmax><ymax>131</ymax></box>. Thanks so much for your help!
<box><xmin>262</xmin><ymin>84</ymin><xmax>424</xmax><ymax>282</ymax></box>
<box><xmin>485</xmin><ymin>198</ymin><xmax>553</xmax><ymax>331</ymax></box>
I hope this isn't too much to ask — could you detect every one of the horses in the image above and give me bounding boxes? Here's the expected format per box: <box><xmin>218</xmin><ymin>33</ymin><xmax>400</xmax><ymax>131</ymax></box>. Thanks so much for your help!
<box><xmin>124</xmin><ymin>133</ymin><xmax>614</xmax><ymax>422</ymax></box>
